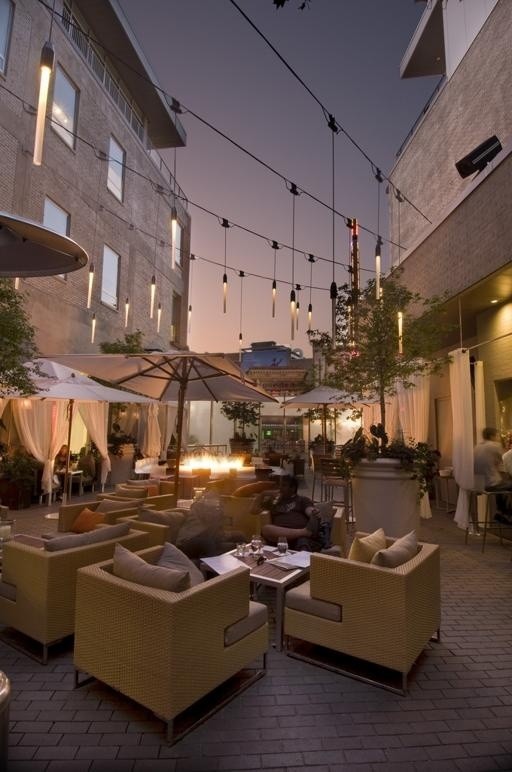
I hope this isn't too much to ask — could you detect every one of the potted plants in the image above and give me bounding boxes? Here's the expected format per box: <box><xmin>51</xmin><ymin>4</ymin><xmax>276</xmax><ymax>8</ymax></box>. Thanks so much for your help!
<box><xmin>220</xmin><ymin>399</ymin><xmax>263</xmax><ymax>463</ymax></box>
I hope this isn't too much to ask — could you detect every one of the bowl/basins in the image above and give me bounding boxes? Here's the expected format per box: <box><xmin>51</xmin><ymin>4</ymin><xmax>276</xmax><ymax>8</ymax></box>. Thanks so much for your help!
<box><xmin>439</xmin><ymin>469</ymin><xmax>451</xmax><ymax>477</ymax></box>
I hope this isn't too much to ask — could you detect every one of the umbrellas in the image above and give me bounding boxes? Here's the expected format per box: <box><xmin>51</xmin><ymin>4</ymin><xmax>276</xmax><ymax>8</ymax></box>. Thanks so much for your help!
<box><xmin>33</xmin><ymin>348</ymin><xmax>279</xmax><ymax>506</ymax></box>
<box><xmin>1</xmin><ymin>370</ymin><xmax>161</xmax><ymax>506</ymax></box>
<box><xmin>282</xmin><ymin>384</ymin><xmax>376</xmax><ymax>455</ymax></box>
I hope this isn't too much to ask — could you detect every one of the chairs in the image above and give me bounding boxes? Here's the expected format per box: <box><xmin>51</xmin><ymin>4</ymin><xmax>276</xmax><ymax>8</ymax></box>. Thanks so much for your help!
<box><xmin>465</xmin><ymin>475</ymin><xmax>511</xmax><ymax>554</ymax></box>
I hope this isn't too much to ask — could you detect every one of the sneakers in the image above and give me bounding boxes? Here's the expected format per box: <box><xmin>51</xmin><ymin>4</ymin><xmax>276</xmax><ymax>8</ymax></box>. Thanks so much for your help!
<box><xmin>307</xmin><ymin>511</ymin><xmax>319</xmax><ymax>540</ymax></box>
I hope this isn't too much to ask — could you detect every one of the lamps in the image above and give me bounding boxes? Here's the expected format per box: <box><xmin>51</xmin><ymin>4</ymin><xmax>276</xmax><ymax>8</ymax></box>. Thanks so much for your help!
<box><xmin>33</xmin><ymin>0</ymin><xmax>55</xmax><ymax>167</ymax></box>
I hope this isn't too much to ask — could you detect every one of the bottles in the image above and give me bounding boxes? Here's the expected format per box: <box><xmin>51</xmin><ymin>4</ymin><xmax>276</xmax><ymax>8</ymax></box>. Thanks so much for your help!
<box><xmin>72</xmin><ymin>461</ymin><xmax>78</xmax><ymax>472</ymax></box>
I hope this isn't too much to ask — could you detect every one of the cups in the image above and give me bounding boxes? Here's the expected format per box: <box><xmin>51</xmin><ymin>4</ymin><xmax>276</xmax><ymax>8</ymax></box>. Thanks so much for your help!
<box><xmin>235</xmin><ymin>534</ymin><xmax>288</xmax><ymax>563</ymax></box>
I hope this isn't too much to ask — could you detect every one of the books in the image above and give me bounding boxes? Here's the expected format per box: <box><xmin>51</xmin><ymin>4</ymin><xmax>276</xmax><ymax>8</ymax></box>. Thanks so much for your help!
<box><xmin>264</xmin><ymin>549</ymin><xmax>314</xmax><ymax>571</ymax></box>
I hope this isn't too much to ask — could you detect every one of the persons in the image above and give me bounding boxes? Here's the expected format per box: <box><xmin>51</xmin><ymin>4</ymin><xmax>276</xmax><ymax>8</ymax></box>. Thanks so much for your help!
<box><xmin>473</xmin><ymin>426</ymin><xmax>512</xmax><ymax>527</ymax></box>
<box><xmin>249</xmin><ymin>474</ymin><xmax>321</xmax><ymax>554</ymax></box>
<box><xmin>54</xmin><ymin>444</ymin><xmax>74</xmax><ymax>497</ymax></box>
